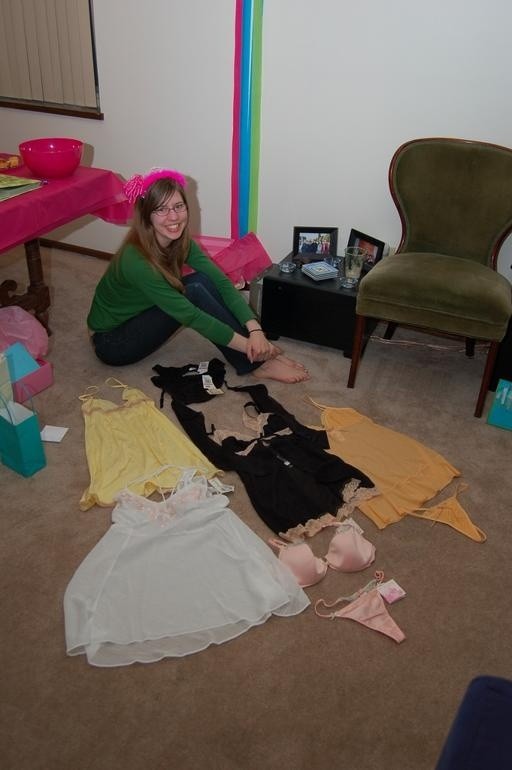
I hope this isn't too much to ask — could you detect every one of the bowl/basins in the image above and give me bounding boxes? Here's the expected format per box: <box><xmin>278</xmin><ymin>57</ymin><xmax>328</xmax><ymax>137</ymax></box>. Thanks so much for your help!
<box><xmin>19</xmin><ymin>138</ymin><xmax>85</xmax><ymax>177</ymax></box>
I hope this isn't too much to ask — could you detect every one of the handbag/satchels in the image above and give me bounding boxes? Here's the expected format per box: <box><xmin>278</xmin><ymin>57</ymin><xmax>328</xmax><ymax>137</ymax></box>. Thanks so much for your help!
<box><xmin>0</xmin><ymin>381</ymin><xmax>47</xmax><ymax>477</ymax></box>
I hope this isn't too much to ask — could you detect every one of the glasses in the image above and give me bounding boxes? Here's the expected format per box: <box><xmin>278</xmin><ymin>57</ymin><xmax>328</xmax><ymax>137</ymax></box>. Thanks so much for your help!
<box><xmin>152</xmin><ymin>203</ymin><xmax>186</xmax><ymax>216</ymax></box>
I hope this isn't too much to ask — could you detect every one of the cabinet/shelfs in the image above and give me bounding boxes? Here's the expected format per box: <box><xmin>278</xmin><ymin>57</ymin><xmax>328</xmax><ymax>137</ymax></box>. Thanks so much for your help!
<box><xmin>261</xmin><ymin>250</ymin><xmax>380</xmax><ymax>359</ymax></box>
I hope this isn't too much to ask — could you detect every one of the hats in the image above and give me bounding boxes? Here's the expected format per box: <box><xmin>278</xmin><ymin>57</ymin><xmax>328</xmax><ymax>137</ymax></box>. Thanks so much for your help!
<box><xmin>123</xmin><ymin>171</ymin><xmax>184</xmax><ymax>204</ymax></box>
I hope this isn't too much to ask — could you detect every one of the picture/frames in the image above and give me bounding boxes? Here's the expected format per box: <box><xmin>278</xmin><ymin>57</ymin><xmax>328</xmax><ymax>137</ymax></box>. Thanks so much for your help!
<box><xmin>293</xmin><ymin>227</ymin><xmax>337</xmax><ymax>262</ymax></box>
<box><xmin>344</xmin><ymin>229</ymin><xmax>385</xmax><ymax>276</ymax></box>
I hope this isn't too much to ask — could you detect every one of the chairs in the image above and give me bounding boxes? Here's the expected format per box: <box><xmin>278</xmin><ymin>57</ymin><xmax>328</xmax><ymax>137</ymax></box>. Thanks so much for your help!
<box><xmin>347</xmin><ymin>138</ymin><xmax>512</xmax><ymax>418</ymax></box>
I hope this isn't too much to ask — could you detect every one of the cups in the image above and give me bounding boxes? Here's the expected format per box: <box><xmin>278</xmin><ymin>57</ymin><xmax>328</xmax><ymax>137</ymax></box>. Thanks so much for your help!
<box><xmin>339</xmin><ymin>246</ymin><xmax>366</xmax><ymax>289</ymax></box>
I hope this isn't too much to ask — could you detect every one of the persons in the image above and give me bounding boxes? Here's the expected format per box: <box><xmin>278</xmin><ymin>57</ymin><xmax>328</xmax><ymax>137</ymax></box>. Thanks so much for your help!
<box><xmin>86</xmin><ymin>169</ymin><xmax>312</xmax><ymax>385</ymax></box>
<box><xmin>366</xmin><ymin>254</ymin><xmax>374</xmax><ymax>266</ymax></box>
<box><xmin>299</xmin><ymin>233</ymin><xmax>331</xmax><ymax>255</ymax></box>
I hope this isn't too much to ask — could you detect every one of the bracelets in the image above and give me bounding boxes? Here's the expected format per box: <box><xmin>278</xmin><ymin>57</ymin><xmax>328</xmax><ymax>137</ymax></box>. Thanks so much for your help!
<box><xmin>247</xmin><ymin>328</ymin><xmax>264</xmax><ymax>335</ymax></box>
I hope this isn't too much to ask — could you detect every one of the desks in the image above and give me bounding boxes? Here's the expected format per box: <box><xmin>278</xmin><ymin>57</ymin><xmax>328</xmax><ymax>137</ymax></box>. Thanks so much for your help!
<box><xmin>0</xmin><ymin>152</ymin><xmax>129</xmax><ymax>337</ymax></box>
